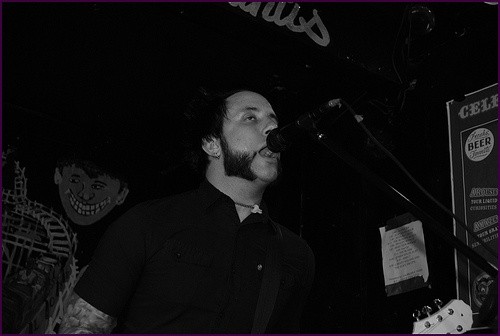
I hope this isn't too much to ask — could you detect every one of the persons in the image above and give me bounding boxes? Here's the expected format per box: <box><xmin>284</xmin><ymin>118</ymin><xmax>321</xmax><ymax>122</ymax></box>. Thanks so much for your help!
<box><xmin>58</xmin><ymin>88</ymin><xmax>315</xmax><ymax>335</ymax></box>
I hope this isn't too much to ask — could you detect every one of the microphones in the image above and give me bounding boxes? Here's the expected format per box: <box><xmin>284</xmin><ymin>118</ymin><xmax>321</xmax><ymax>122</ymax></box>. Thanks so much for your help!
<box><xmin>266</xmin><ymin>99</ymin><xmax>343</xmax><ymax>153</ymax></box>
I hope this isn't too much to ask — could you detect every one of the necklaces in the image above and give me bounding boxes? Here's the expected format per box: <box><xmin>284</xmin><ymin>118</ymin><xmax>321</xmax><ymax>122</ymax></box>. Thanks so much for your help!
<box><xmin>235</xmin><ymin>201</ymin><xmax>263</xmax><ymax>215</ymax></box>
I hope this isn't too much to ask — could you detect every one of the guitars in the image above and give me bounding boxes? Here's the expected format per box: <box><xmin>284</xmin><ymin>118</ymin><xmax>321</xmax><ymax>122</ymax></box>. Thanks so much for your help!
<box><xmin>411</xmin><ymin>298</ymin><xmax>473</xmax><ymax>334</ymax></box>
